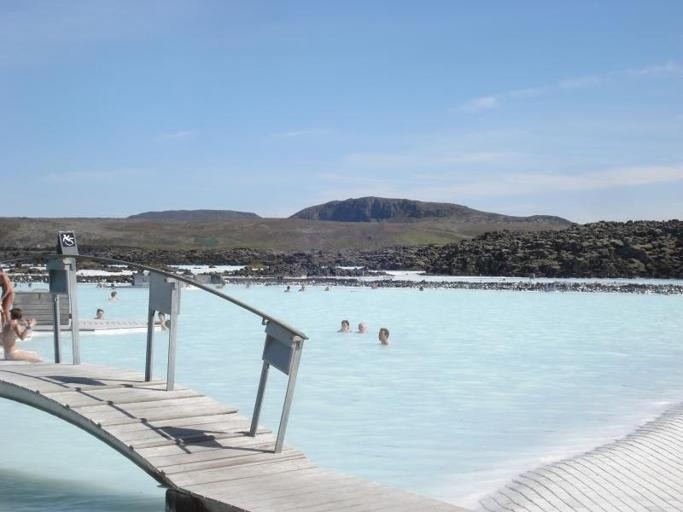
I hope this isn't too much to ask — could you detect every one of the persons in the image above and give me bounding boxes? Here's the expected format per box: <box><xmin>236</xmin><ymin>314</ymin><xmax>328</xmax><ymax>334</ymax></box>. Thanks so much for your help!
<box><xmin>284</xmin><ymin>286</ymin><xmax>292</xmax><ymax>292</ymax></box>
<box><xmin>0</xmin><ymin>267</ymin><xmax>15</xmax><ymax>326</ymax></box>
<box><xmin>378</xmin><ymin>328</ymin><xmax>390</xmax><ymax>346</ymax></box>
<box><xmin>358</xmin><ymin>322</ymin><xmax>368</xmax><ymax>333</ymax></box>
<box><xmin>338</xmin><ymin>320</ymin><xmax>352</xmax><ymax>335</ymax></box>
<box><xmin>156</xmin><ymin>313</ymin><xmax>170</xmax><ymax>331</ymax></box>
<box><xmin>0</xmin><ymin>307</ymin><xmax>43</xmax><ymax>362</ymax></box>
<box><xmin>94</xmin><ymin>307</ymin><xmax>106</xmax><ymax>321</ymax></box>
<box><xmin>109</xmin><ymin>292</ymin><xmax>119</xmax><ymax>302</ymax></box>
